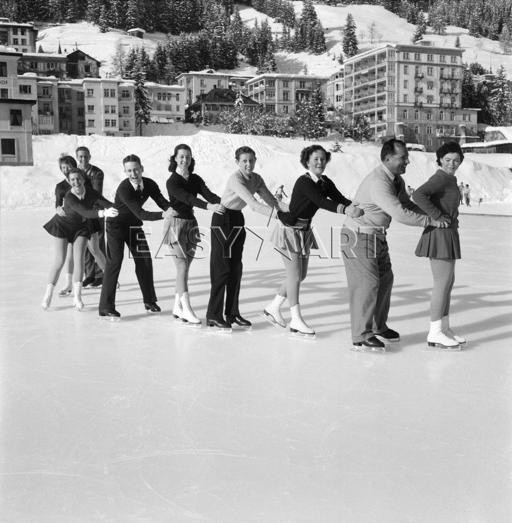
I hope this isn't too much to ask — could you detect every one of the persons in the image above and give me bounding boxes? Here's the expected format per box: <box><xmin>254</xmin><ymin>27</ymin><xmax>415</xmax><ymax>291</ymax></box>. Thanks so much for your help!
<box><xmin>413</xmin><ymin>140</ymin><xmax>467</xmax><ymax>348</ymax></box>
<box><xmin>275</xmin><ymin>184</ymin><xmax>287</xmax><ymax>202</ymax></box>
<box><xmin>339</xmin><ymin>139</ymin><xmax>448</xmax><ymax>348</ymax></box>
<box><xmin>264</xmin><ymin>144</ymin><xmax>365</xmax><ymax>333</ymax></box>
<box><xmin>407</xmin><ymin>185</ymin><xmax>414</xmax><ymax>198</ymax></box>
<box><xmin>41</xmin><ymin>147</ymin><xmax>119</xmax><ymax>308</ymax></box>
<box><xmin>166</xmin><ymin>144</ymin><xmax>227</xmax><ymax>325</ymax></box>
<box><xmin>206</xmin><ymin>146</ymin><xmax>297</xmax><ymax>327</ymax></box>
<box><xmin>458</xmin><ymin>182</ymin><xmax>470</xmax><ymax>207</ymax></box>
<box><xmin>100</xmin><ymin>154</ymin><xmax>180</xmax><ymax>317</ymax></box>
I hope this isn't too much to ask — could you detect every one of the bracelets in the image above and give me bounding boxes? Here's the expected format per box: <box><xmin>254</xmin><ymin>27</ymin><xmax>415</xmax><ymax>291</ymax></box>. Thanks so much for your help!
<box><xmin>342</xmin><ymin>206</ymin><xmax>347</xmax><ymax>215</ymax></box>
